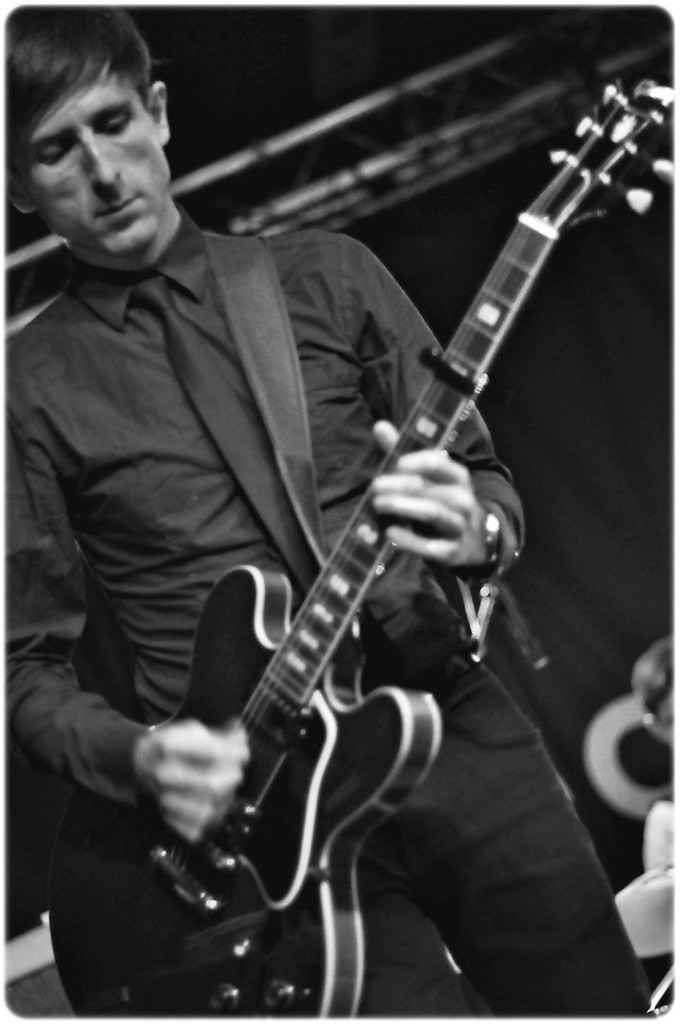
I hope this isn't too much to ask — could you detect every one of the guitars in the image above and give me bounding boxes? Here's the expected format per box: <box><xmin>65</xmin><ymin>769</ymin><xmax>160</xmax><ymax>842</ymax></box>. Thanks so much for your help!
<box><xmin>47</xmin><ymin>75</ymin><xmax>675</xmax><ymax>1018</ymax></box>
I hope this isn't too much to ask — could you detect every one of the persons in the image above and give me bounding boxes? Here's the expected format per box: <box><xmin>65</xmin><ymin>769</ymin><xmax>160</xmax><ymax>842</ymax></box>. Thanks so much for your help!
<box><xmin>5</xmin><ymin>5</ymin><xmax>651</xmax><ymax>1017</ymax></box>
<box><xmin>630</xmin><ymin>633</ymin><xmax>675</xmax><ymax>873</ymax></box>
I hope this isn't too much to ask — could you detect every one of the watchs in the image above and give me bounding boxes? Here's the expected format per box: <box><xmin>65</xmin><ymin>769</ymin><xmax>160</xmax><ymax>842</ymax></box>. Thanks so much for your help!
<box><xmin>479</xmin><ymin>513</ymin><xmax>501</xmax><ymax>564</ymax></box>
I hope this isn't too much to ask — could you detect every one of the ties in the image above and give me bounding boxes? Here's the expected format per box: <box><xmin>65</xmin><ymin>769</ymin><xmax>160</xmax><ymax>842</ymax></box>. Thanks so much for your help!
<box><xmin>130</xmin><ymin>277</ymin><xmax>322</xmax><ymax>598</ymax></box>
